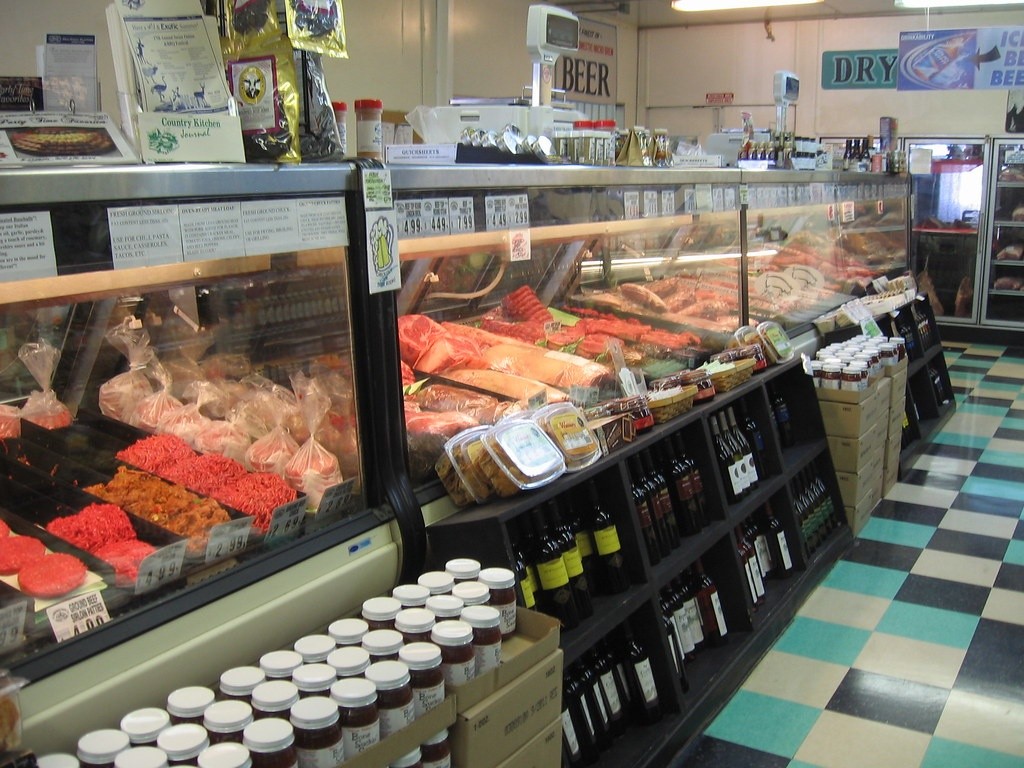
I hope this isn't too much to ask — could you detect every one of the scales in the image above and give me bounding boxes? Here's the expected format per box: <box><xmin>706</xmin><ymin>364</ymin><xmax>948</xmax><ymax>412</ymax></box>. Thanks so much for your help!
<box><xmin>707</xmin><ymin>71</ymin><xmax>801</xmax><ymax>167</ymax></box>
<box><xmin>428</xmin><ymin>3</ymin><xmax>589</xmax><ymax>146</ymax></box>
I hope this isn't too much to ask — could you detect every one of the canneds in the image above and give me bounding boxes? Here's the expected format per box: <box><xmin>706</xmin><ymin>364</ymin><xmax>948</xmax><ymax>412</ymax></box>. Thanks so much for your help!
<box><xmin>811</xmin><ymin>334</ymin><xmax>907</xmax><ymax>391</ymax></box>
<box><xmin>36</xmin><ymin>557</ymin><xmax>516</xmax><ymax>768</ymax></box>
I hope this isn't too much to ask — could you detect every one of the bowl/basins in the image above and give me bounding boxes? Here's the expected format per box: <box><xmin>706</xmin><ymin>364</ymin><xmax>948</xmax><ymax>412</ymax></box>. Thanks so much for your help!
<box><xmin>435</xmin><ymin>402</ymin><xmax>602</xmax><ymax>507</ymax></box>
<box><xmin>0</xmin><ymin>664</ymin><xmax>31</xmax><ymax>756</ymax></box>
<box><xmin>727</xmin><ymin>320</ymin><xmax>796</xmax><ymax>366</ymax></box>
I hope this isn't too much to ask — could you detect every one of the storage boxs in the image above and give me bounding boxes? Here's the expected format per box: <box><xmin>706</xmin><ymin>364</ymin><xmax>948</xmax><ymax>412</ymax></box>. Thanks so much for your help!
<box><xmin>330</xmin><ymin>289</ymin><xmax>959</xmax><ymax>768</ymax></box>
<box><xmin>0</xmin><ymin>409</ymin><xmax>307</xmax><ymax>655</ymax></box>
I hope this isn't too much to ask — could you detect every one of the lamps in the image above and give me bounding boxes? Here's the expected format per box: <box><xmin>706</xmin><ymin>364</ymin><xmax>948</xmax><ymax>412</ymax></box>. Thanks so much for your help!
<box><xmin>671</xmin><ymin>0</ymin><xmax>825</xmax><ymax>12</ymax></box>
<box><xmin>895</xmin><ymin>0</ymin><xmax>1024</xmax><ymax>8</ymax></box>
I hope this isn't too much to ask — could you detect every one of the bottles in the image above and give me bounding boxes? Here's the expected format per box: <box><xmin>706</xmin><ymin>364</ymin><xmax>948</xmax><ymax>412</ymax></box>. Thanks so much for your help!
<box><xmin>553</xmin><ymin>120</ymin><xmax>617</xmax><ymax>168</ymax></box>
<box><xmin>591</xmin><ymin>343</ymin><xmax>768</xmax><ymax>433</ymax></box>
<box><xmin>354</xmin><ymin>99</ymin><xmax>384</xmax><ymax>159</ymax></box>
<box><xmin>507</xmin><ymin>480</ymin><xmax>632</xmax><ymax>632</ymax></box>
<box><xmin>559</xmin><ymin>625</ymin><xmax>663</xmax><ymax>768</ymax></box>
<box><xmin>657</xmin><ymin>556</ymin><xmax>728</xmax><ymax>694</ymax></box>
<box><xmin>878</xmin><ymin>304</ymin><xmax>946</xmax><ymax>456</ymax></box>
<box><xmin>625</xmin><ymin>431</ymin><xmax>713</xmax><ymax>567</ymax></box>
<box><xmin>843</xmin><ymin>138</ymin><xmax>871</xmax><ymax>172</ymax></box>
<box><xmin>707</xmin><ymin>376</ymin><xmax>800</xmax><ymax>503</ymax></box>
<box><xmin>330</xmin><ymin>101</ymin><xmax>347</xmax><ymax>152</ymax></box>
<box><xmin>733</xmin><ymin>501</ymin><xmax>793</xmax><ymax>615</ymax></box>
<box><xmin>786</xmin><ymin>459</ymin><xmax>839</xmax><ymax>562</ymax></box>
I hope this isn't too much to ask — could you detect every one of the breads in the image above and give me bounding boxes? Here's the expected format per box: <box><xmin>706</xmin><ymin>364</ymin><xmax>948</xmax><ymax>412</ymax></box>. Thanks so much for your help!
<box><xmin>993</xmin><ymin>172</ymin><xmax>1024</xmax><ymax>293</ymax></box>
